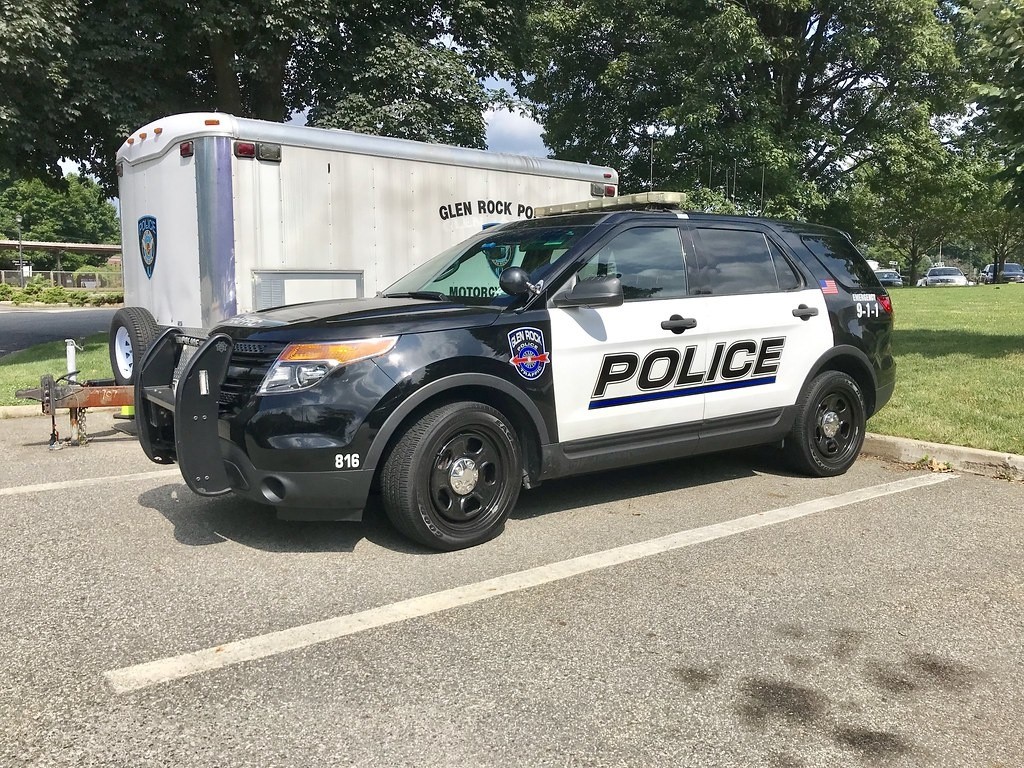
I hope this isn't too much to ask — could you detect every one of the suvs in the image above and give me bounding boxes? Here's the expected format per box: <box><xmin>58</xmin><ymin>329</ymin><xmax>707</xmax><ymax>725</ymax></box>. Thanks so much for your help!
<box><xmin>134</xmin><ymin>193</ymin><xmax>899</xmax><ymax>552</ymax></box>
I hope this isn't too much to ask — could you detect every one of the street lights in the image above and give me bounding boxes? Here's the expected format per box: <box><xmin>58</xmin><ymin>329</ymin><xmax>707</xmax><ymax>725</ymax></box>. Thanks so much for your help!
<box><xmin>969</xmin><ymin>246</ymin><xmax>974</xmax><ymax>282</ymax></box>
<box><xmin>16</xmin><ymin>212</ymin><xmax>26</xmax><ymax>291</ymax></box>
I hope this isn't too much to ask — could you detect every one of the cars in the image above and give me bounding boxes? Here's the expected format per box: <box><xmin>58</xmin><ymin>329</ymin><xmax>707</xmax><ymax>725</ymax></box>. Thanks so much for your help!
<box><xmin>925</xmin><ymin>265</ymin><xmax>969</xmax><ymax>287</ymax></box>
<box><xmin>980</xmin><ymin>264</ymin><xmax>998</xmax><ymax>283</ymax></box>
<box><xmin>984</xmin><ymin>262</ymin><xmax>1024</xmax><ymax>285</ymax></box>
<box><xmin>872</xmin><ymin>269</ymin><xmax>907</xmax><ymax>288</ymax></box>
